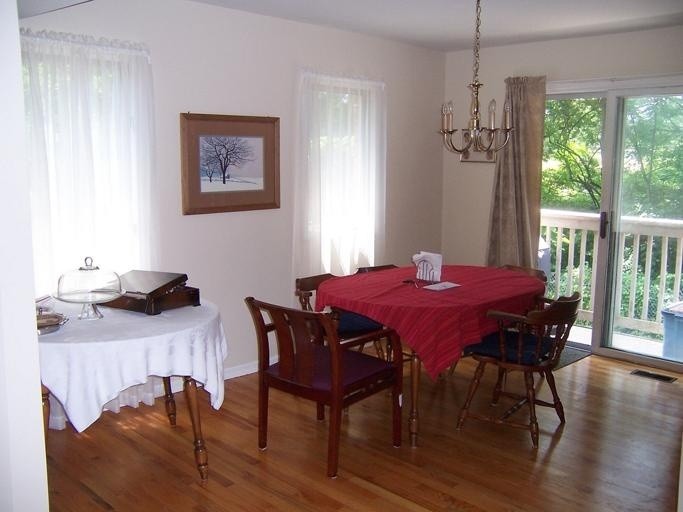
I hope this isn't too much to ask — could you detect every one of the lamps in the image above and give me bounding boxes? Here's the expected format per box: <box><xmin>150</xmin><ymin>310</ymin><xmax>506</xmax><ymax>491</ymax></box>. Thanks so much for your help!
<box><xmin>439</xmin><ymin>0</ymin><xmax>515</xmax><ymax>154</ymax></box>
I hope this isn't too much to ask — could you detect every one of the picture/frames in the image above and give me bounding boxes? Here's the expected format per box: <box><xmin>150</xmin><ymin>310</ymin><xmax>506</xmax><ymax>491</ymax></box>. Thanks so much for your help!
<box><xmin>460</xmin><ymin>129</ymin><xmax>496</xmax><ymax>163</ymax></box>
<box><xmin>180</xmin><ymin>113</ymin><xmax>280</xmax><ymax>215</ymax></box>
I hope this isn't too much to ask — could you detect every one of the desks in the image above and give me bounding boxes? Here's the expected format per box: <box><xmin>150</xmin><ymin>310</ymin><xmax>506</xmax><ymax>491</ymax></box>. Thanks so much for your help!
<box><xmin>38</xmin><ymin>298</ymin><xmax>228</xmax><ymax>479</ymax></box>
